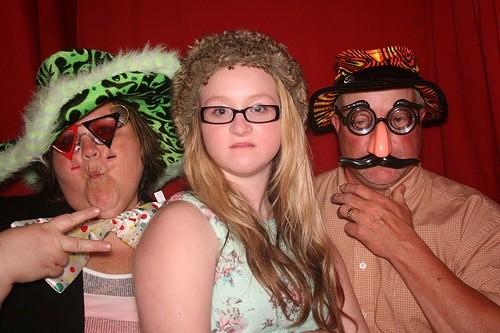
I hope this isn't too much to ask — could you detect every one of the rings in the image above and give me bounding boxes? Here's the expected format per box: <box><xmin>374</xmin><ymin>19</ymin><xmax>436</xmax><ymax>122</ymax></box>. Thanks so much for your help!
<box><xmin>346</xmin><ymin>207</ymin><xmax>354</xmax><ymax>221</ymax></box>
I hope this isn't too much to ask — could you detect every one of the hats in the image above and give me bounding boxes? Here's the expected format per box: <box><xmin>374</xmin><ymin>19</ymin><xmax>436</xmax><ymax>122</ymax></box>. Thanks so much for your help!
<box><xmin>0</xmin><ymin>38</ymin><xmax>185</xmax><ymax>200</ymax></box>
<box><xmin>169</xmin><ymin>29</ymin><xmax>308</xmax><ymax>155</ymax></box>
<box><xmin>308</xmin><ymin>44</ymin><xmax>448</xmax><ymax>137</ymax></box>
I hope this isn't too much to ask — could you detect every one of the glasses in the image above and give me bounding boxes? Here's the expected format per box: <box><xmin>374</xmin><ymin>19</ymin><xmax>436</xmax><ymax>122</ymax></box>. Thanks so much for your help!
<box><xmin>333</xmin><ymin>98</ymin><xmax>426</xmax><ymax>135</ymax></box>
<box><xmin>49</xmin><ymin>112</ymin><xmax>119</xmax><ymax>161</ymax></box>
<box><xmin>200</xmin><ymin>104</ymin><xmax>281</xmax><ymax>125</ymax></box>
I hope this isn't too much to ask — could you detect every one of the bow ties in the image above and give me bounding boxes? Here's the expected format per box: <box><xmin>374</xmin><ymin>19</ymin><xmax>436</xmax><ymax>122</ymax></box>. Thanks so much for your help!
<box><xmin>10</xmin><ymin>200</ymin><xmax>162</xmax><ymax>293</ymax></box>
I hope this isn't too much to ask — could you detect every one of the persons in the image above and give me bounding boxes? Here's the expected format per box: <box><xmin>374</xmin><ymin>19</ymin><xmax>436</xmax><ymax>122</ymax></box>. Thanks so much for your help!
<box><xmin>307</xmin><ymin>45</ymin><xmax>500</xmax><ymax>333</ymax></box>
<box><xmin>1</xmin><ymin>47</ymin><xmax>190</xmax><ymax>333</ymax></box>
<box><xmin>134</xmin><ymin>30</ymin><xmax>369</xmax><ymax>333</ymax></box>
